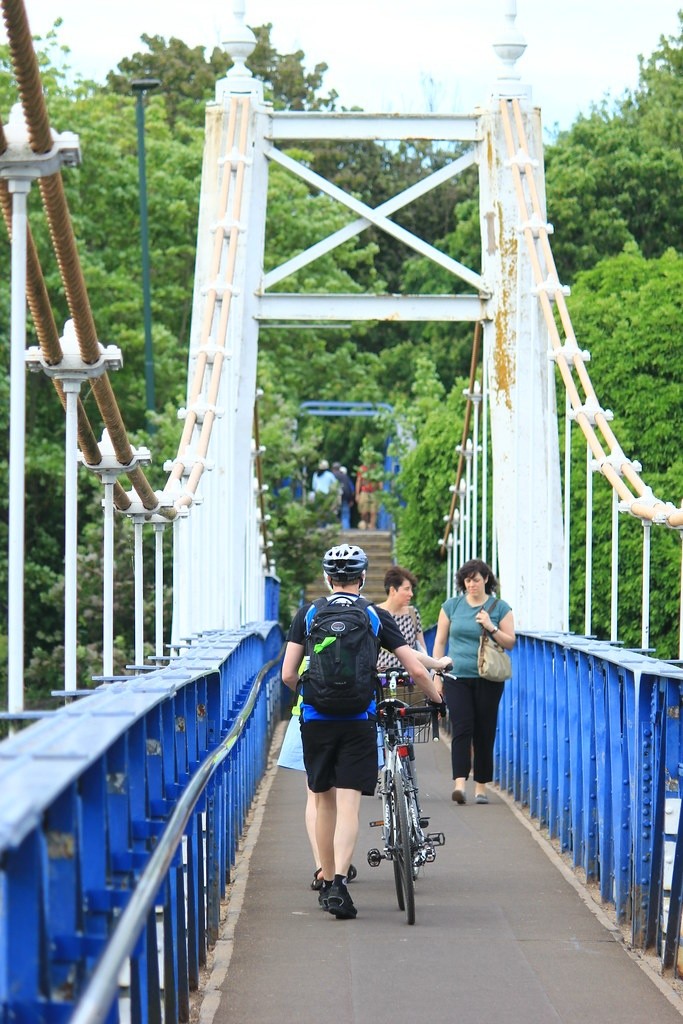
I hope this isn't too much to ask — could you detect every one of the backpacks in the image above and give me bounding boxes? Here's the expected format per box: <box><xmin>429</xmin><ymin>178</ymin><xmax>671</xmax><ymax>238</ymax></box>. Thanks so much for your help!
<box><xmin>293</xmin><ymin>596</ymin><xmax>384</xmax><ymax>715</ymax></box>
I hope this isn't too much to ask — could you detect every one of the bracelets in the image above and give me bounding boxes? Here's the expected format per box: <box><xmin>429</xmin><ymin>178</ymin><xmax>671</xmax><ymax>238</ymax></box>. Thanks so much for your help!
<box><xmin>433</xmin><ymin>674</ymin><xmax>444</xmax><ymax>682</ymax></box>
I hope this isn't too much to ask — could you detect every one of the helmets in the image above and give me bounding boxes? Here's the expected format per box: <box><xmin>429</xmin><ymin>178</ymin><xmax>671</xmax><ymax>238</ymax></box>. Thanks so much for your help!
<box><xmin>322</xmin><ymin>543</ymin><xmax>369</xmax><ymax>582</ymax></box>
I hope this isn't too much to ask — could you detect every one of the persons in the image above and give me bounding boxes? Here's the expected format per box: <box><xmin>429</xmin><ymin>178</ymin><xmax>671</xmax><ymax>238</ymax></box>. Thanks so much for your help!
<box><xmin>331</xmin><ymin>461</ymin><xmax>355</xmax><ymax>531</ymax></box>
<box><xmin>277</xmin><ymin>646</ymin><xmax>452</xmax><ymax>891</ymax></box>
<box><xmin>339</xmin><ymin>466</ymin><xmax>355</xmax><ymax>497</ymax></box>
<box><xmin>355</xmin><ymin>451</ymin><xmax>385</xmax><ymax>532</ymax></box>
<box><xmin>373</xmin><ymin>565</ymin><xmax>431</xmax><ymax>800</ymax></box>
<box><xmin>312</xmin><ymin>459</ymin><xmax>343</xmax><ymax>536</ymax></box>
<box><xmin>280</xmin><ymin>543</ymin><xmax>447</xmax><ymax>920</ymax></box>
<box><xmin>432</xmin><ymin>558</ymin><xmax>517</xmax><ymax>806</ymax></box>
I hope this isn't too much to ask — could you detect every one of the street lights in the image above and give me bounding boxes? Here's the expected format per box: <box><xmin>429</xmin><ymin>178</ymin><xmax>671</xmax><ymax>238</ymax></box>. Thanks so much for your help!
<box><xmin>131</xmin><ymin>79</ymin><xmax>162</xmax><ymax>433</ymax></box>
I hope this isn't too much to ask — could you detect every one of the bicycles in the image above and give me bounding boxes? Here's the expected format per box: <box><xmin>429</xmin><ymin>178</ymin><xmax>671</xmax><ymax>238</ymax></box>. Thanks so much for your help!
<box><xmin>367</xmin><ymin>663</ymin><xmax>456</xmax><ymax>928</ymax></box>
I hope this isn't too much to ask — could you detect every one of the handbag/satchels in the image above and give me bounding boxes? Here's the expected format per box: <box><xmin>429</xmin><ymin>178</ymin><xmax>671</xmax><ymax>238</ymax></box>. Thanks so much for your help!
<box><xmin>408</xmin><ymin>606</ymin><xmax>432</xmax><ymax>673</ymax></box>
<box><xmin>478</xmin><ymin>598</ymin><xmax>513</xmax><ymax>682</ymax></box>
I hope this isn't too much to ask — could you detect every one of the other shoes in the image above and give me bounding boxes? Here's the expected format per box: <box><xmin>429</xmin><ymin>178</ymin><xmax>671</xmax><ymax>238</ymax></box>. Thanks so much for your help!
<box><xmin>475</xmin><ymin>793</ymin><xmax>489</xmax><ymax>804</ymax></box>
<box><xmin>452</xmin><ymin>790</ymin><xmax>466</xmax><ymax>805</ymax></box>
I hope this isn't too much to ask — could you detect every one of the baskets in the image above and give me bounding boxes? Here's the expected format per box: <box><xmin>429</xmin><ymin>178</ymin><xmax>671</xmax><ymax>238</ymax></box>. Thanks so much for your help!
<box><xmin>376</xmin><ymin>707</ymin><xmax>432</xmax><ymax>744</ymax></box>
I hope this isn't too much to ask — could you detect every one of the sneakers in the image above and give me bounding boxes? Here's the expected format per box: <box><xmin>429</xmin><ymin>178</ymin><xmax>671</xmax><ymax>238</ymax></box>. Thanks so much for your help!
<box><xmin>318</xmin><ymin>874</ymin><xmax>357</xmax><ymax>919</ymax></box>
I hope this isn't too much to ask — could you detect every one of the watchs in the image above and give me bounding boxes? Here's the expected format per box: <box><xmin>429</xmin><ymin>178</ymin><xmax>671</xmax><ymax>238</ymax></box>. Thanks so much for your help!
<box><xmin>490</xmin><ymin>625</ymin><xmax>498</xmax><ymax>635</ymax></box>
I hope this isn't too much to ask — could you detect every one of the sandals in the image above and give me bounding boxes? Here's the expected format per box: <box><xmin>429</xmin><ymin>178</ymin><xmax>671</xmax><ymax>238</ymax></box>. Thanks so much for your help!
<box><xmin>311</xmin><ymin>868</ymin><xmax>324</xmax><ymax>890</ymax></box>
<box><xmin>347</xmin><ymin>864</ymin><xmax>357</xmax><ymax>883</ymax></box>
